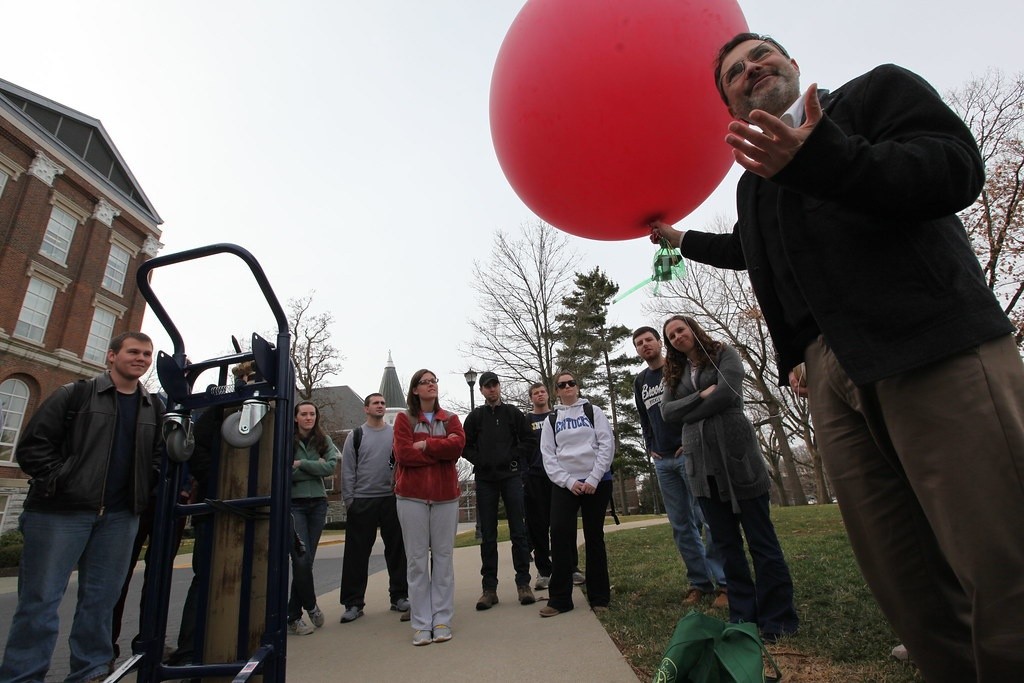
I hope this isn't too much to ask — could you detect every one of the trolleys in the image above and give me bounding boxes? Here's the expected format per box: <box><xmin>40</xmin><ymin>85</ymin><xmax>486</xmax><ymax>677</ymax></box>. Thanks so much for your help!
<box><xmin>95</xmin><ymin>242</ymin><xmax>297</xmax><ymax>683</ymax></box>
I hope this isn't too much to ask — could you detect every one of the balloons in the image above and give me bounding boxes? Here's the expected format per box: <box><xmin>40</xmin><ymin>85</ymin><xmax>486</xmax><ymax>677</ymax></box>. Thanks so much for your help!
<box><xmin>488</xmin><ymin>0</ymin><xmax>750</xmax><ymax>241</ymax></box>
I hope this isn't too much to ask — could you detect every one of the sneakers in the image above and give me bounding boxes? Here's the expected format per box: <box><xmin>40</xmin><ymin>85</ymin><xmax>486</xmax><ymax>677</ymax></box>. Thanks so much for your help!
<box><xmin>680</xmin><ymin>585</ymin><xmax>703</xmax><ymax>606</ymax></box>
<box><xmin>288</xmin><ymin>615</ymin><xmax>313</xmax><ymax>635</ymax></box>
<box><xmin>390</xmin><ymin>599</ymin><xmax>411</xmax><ymax>612</ymax></box>
<box><xmin>710</xmin><ymin>585</ymin><xmax>729</xmax><ymax>610</ymax></box>
<box><xmin>517</xmin><ymin>583</ymin><xmax>536</xmax><ymax>605</ymax></box>
<box><xmin>573</xmin><ymin>572</ymin><xmax>586</xmax><ymax>584</ymax></box>
<box><xmin>340</xmin><ymin>602</ymin><xmax>364</xmax><ymax>624</ymax></box>
<box><xmin>534</xmin><ymin>570</ymin><xmax>551</xmax><ymax>590</ymax></box>
<box><xmin>432</xmin><ymin>624</ymin><xmax>452</xmax><ymax>642</ymax></box>
<box><xmin>475</xmin><ymin>586</ymin><xmax>498</xmax><ymax>610</ymax></box>
<box><xmin>307</xmin><ymin>604</ymin><xmax>325</xmax><ymax>627</ymax></box>
<box><xmin>411</xmin><ymin>629</ymin><xmax>431</xmax><ymax>645</ymax></box>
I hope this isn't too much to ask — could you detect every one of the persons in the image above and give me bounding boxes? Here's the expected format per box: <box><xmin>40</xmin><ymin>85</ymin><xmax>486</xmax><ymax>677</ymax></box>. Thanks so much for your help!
<box><xmin>633</xmin><ymin>327</ymin><xmax>727</xmax><ymax>608</ymax></box>
<box><xmin>527</xmin><ymin>382</ymin><xmax>584</xmax><ymax>590</ymax></box>
<box><xmin>539</xmin><ymin>369</ymin><xmax>615</xmax><ymax>616</ymax></box>
<box><xmin>340</xmin><ymin>393</ymin><xmax>409</xmax><ymax>621</ymax></box>
<box><xmin>1</xmin><ymin>331</ymin><xmax>160</xmax><ymax>683</ymax></box>
<box><xmin>289</xmin><ymin>401</ymin><xmax>338</xmax><ymax>634</ymax></box>
<box><xmin>394</xmin><ymin>369</ymin><xmax>466</xmax><ymax>645</ymax></box>
<box><xmin>660</xmin><ymin>316</ymin><xmax>800</xmax><ymax>643</ymax></box>
<box><xmin>461</xmin><ymin>371</ymin><xmax>536</xmax><ymax>609</ymax></box>
<box><xmin>650</xmin><ymin>33</ymin><xmax>1024</xmax><ymax>682</ymax></box>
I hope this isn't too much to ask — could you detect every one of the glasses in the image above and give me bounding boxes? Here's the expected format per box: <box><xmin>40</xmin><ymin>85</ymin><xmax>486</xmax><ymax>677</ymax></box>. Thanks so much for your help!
<box><xmin>717</xmin><ymin>36</ymin><xmax>791</xmax><ymax>97</ymax></box>
<box><xmin>556</xmin><ymin>379</ymin><xmax>578</xmax><ymax>390</ymax></box>
<box><xmin>417</xmin><ymin>378</ymin><xmax>439</xmax><ymax>386</ymax></box>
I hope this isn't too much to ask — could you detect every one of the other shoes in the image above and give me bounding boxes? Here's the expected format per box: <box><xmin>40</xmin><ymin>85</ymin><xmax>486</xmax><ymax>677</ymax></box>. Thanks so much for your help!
<box><xmin>162</xmin><ymin>643</ymin><xmax>176</xmax><ymax>662</ymax></box>
<box><xmin>84</xmin><ymin>675</ymin><xmax>101</xmax><ymax>683</ymax></box>
<box><xmin>890</xmin><ymin>644</ymin><xmax>912</xmax><ymax>660</ymax></box>
<box><xmin>592</xmin><ymin>606</ymin><xmax>608</xmax><ymax>613</ymax></box>
<box><xmin>107</xmin><ymin>658</ymin><xmax>117</xmax><ymax>674</ymax></box>
<box><xmin>399</xmin><ymin>608</ymin><xmax>412</xmax><ymax>621</ymax></box>
<box><xmin>539</xmin><ymin>606</ymin><xmax>559</xmax><ymax>616</ymax></box>
<box><xmin>759</xmin><ymin>630</ymin><xmax>780</xmax><ymax>642</ymax></box>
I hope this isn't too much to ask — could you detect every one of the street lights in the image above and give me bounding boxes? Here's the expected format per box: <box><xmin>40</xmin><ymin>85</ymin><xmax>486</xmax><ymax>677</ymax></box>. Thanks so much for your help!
<box><xmin>463</xmin><ymin>367</ymin><xmax>483</xmax><ymax>538</ymax></box>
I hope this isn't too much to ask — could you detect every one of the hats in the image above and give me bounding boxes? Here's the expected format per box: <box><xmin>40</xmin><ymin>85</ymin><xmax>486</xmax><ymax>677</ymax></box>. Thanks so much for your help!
<box><xmin>479</xmin><ymin>372</ymin><xmax>500</xmax><ymax>389</ymax></box>
